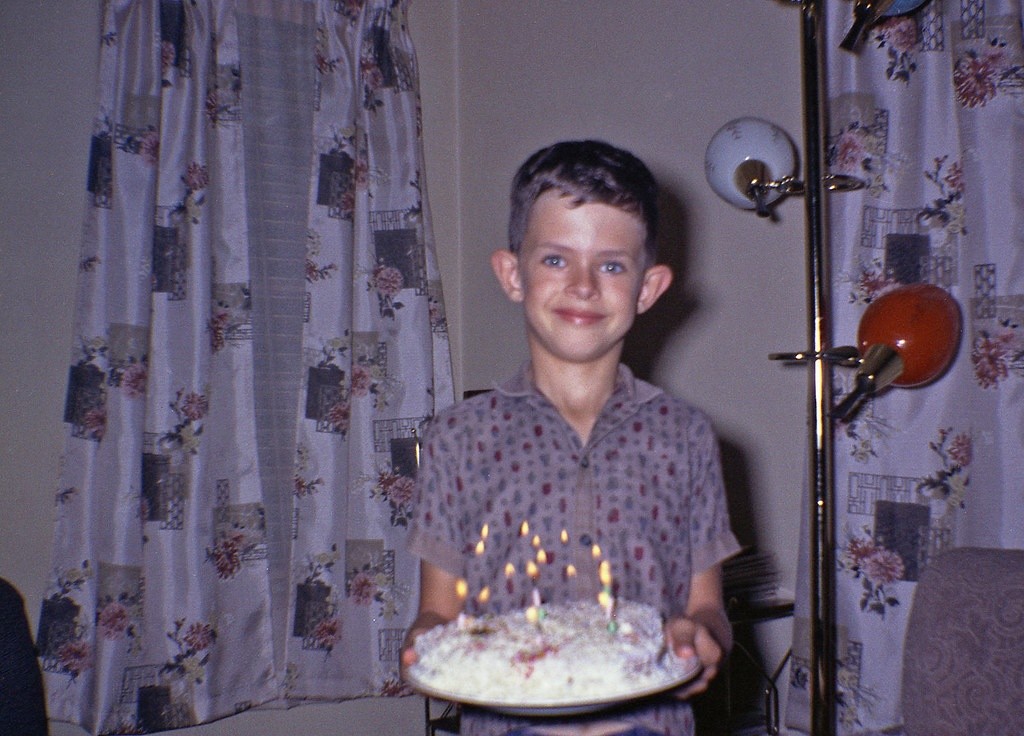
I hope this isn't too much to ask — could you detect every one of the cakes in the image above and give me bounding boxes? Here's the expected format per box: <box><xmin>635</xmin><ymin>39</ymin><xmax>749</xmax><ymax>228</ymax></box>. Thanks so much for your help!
<box><xmin>413</xmin><ymin>597</ymin><xmax>666</xmax><ymax>699</ymax></box>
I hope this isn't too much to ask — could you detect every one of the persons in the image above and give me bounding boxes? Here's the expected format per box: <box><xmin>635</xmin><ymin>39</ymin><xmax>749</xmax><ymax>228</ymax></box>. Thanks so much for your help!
<box><xmin>399</xmin><ymin>138</ymin><xmax>746</xmax><ymax>735</ymax></box>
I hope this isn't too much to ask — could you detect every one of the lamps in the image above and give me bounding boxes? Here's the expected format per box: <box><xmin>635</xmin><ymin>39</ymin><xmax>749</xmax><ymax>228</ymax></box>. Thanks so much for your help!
<box><xmin>704</xmin><ymin>0</ymin><xmax>962</xmax><ymax>736</ymax></box>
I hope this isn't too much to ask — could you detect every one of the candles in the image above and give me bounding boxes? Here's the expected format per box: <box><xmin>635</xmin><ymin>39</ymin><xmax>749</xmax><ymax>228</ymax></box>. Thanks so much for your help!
<box><xmin>457</xmin><ymin>521</ymin><xmax>614</xmax><ymax>634</ymax></box>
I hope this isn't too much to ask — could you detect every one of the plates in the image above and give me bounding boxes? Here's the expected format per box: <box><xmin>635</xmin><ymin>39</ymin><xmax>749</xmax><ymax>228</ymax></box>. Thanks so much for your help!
<box><xmin>402</xmin><ymin>656</ymin><xmax>705</xmax><ymax>717</ymax></box>
